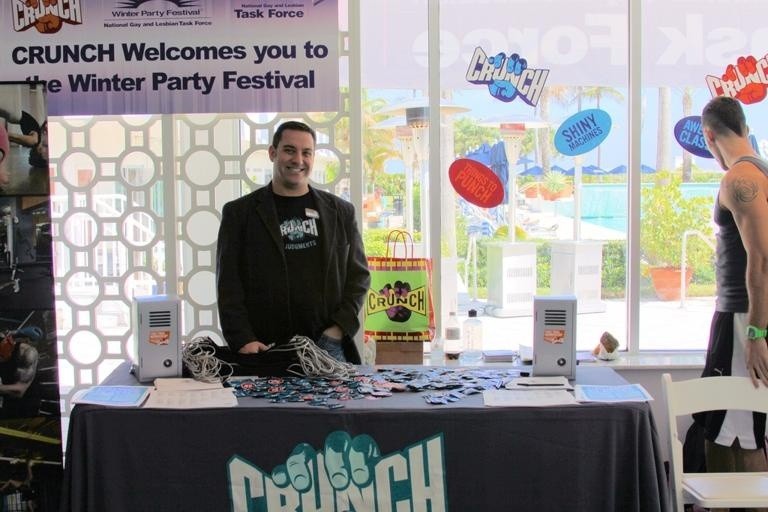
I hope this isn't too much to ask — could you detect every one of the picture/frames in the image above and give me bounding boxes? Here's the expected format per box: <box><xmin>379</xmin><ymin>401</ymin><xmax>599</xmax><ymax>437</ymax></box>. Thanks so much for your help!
<box><xmin>59</xmin><ymin>362</ymin><xmax>674</xmax><ymax>512</ymax></box>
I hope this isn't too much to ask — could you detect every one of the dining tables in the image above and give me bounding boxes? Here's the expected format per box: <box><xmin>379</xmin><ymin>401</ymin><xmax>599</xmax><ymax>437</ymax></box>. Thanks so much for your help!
<box><xmin>525</xmin><ymin>181</ymin><xmax>537</xmax><ymax>199</ymax></box>
<box><xmin>542</xmin><ymin>169</ymin><xmax>573</xmax><ymax>202</ymax></box>
<box><xmin>637</xmin><ymin>170</ymin><xmax>716</xmax><ymax>302</ymax></box>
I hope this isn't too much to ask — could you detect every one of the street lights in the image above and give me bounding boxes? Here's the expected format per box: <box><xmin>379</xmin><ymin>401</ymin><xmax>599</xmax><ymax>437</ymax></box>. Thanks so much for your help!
<box><xmin>362</xmin><ymin>255</ymin><xmax>436</xmax><ymax>343</ymax></box>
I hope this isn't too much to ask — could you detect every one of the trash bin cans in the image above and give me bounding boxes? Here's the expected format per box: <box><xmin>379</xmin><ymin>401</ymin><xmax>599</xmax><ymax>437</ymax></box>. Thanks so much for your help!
<box><xmin>745</xmin><ymin>324</ymin><xmax>767</xmax><ymax>341</ymax></box>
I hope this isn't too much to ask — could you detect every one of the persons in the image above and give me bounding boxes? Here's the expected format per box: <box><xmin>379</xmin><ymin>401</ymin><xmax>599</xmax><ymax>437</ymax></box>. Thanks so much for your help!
<box><xmin>0</xmin><ymin>327</ymin><xmax>41</xmax><ymax>417</ymax></box>
<box><xmin>1</xmin><ymin>458</ymin><xmax>37</xmax><ymax>511</ymax></box>
<box><xmin>691</xmin><ymin>95</ymin><xmax>768</xmax><ymax>512</ymax></box>
<box><xmin>217</xmin><ymin>122</ymin><xmax>370</xmax><ymax>366</ymax></box>
<box><xmin>0</xmin><ymin>122</ymin><xmax>12</xmax><ymax>190</ymax></box>
<box><xmin>0</xmin><ymin>103</ymin><xmax>48</xmax><ymax>168</ymax></box>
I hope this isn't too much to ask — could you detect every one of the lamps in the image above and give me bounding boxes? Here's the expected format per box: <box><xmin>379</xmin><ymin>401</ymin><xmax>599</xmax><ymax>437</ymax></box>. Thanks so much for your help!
<box><xmin>662</xmin><ymin>372</ymin><xmax>768</xmax><ymax>512</ymax></box>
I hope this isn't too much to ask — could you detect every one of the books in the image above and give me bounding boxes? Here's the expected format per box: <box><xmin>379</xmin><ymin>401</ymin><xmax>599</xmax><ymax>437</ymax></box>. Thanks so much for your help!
<box><xmin>374</xmin><ymin>341</ymin><xmax>423</xmax><ymax>364</ymax></box>
